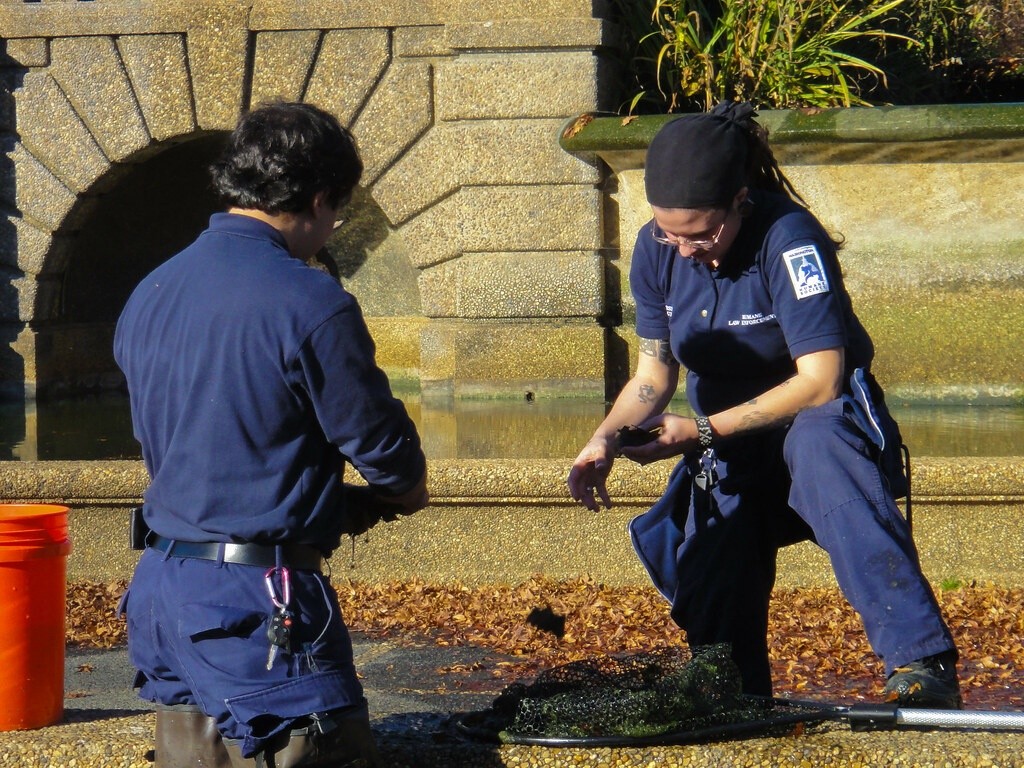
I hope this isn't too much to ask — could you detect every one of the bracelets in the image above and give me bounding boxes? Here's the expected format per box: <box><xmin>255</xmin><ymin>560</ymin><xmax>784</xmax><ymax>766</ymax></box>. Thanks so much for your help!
<box><xmin>693</xmin><ymin>416</ymin><xmax>713</xmax><ymax>448</ymax></box>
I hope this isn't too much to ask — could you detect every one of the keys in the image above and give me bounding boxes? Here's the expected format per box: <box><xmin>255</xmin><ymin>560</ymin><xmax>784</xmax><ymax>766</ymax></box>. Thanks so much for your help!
<box><xmin>267</xmin><ymin>603</ymin><xmax>296</xmax><ymax>672</ymax></box>
<box><xmin>695</xmin><ymin>468</ymin><xmax>714</xmax><ymax>511</ymax></box>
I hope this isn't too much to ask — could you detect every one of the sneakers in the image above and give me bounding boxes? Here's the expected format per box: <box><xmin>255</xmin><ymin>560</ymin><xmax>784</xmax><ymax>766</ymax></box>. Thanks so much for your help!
<box><xmin>883</xmin><ymin>652</ymin><xmax>965</xmax><ymax>709</ymax></box>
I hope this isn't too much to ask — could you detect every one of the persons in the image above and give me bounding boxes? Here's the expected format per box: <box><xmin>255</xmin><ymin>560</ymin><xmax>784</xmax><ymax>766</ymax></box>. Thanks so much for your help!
<box><xmin>112</xmin><ymin>102</ymin><xmax>431</xmax><ymax>768</ymax></box>
<box><xmin>566</xmin><ymin>100</ymin><xmax>965</xmax><ymax>706</ymax></box>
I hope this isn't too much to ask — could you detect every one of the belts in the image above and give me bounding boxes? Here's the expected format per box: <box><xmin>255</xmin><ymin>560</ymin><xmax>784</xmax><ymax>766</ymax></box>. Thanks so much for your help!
<box><xmin>149</xmin><ymin>533</ymin><xmax>323</xmax><ymax>570</ymax></box>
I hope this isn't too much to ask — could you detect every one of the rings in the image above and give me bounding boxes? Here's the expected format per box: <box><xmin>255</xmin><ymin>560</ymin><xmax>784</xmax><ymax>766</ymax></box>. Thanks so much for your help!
<box><xmin>586</xmin><ymin>487</ymin><xmax>594</xmax><ymax>491</ymax></box>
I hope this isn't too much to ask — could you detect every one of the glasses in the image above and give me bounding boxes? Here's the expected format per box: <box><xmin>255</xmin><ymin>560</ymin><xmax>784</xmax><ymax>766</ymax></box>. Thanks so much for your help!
<box><xmin>651</xmin><ymin>206</ymin><xmax>732</xmax><ymax>249</ymax></box>
<box><xmin>333</xmin><ymin>205</ymin><xmax>350</xmax><ymax>230</ymax></box>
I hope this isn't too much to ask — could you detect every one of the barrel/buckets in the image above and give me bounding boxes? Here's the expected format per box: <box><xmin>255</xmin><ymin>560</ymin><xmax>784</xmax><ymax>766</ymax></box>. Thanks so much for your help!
<box><xmin>0</xmin><ymin>504</ymin><xmax>70</xmax><ymax>731</ymax></box>
